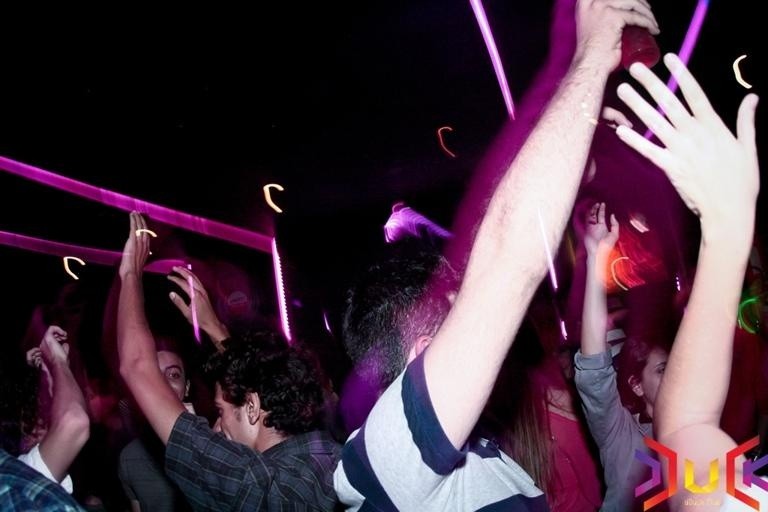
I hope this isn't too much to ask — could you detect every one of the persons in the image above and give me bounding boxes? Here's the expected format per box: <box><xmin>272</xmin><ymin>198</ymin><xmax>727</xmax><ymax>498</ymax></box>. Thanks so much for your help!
<box><xmin>569</xmin><ymin>198</ymin><xmax>669</xmax><ymax>512</ymax></box>
<box><xmin>3</xmin><ymin>177</ymin><xmax>603</xmax><ymax>512</ymax></box>
<box><xmin>329</xmin><ymin>0</ymin><xmax>666</xmax><ymax>512</ymax></box>
<box><xmin>613</xmin><ymin>50</ymin><xmax>768</xmax><ymax>512</ymax></box>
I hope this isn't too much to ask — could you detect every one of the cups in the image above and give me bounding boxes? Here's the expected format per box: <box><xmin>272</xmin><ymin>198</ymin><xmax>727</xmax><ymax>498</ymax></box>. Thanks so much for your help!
<box><xmin>618</xmin><ymin>26</ymin><xmax>660</xmax><ymax>73</ymax></box>
